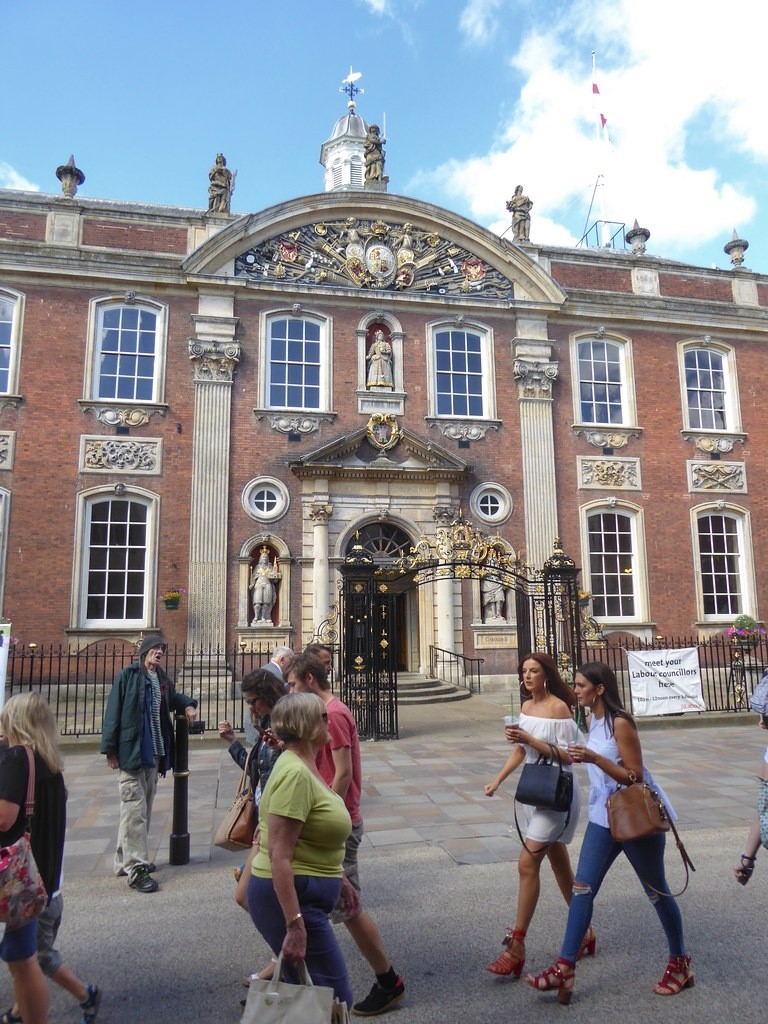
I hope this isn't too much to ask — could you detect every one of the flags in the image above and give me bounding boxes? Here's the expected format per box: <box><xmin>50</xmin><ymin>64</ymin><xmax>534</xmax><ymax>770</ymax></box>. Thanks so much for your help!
<box><xmin>593</xmin><ymin>84</ymin><xmax>600</xmax><ymax>94</ymax></box>
<box><xmin>600</xmin><ymin>114</ymin><xmax>607</xmax><ymax>129</ymax></box>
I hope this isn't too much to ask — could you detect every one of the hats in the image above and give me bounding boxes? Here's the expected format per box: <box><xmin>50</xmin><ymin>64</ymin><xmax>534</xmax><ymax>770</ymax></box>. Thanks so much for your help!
<box><xmin>139</xmin><ymin>634</ymin><xmax>167</xmax><ymax>656</ymax></box>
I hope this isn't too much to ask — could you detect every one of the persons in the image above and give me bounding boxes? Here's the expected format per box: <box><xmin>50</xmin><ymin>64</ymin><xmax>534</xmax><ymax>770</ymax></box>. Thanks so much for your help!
<box><xmin>245</xmin><ymin>645</ymin><xmax>295</xmax><ymax>749</ymax></box>
<box><xmin>481</xmin><ymin>549</ymin><xmax>508</xmax><ymax>618</ymax></box>
<box><xmin>303</xmin><ymin>643</ymin><xmax>333</xmax><ymax>678</ymax></box>
<box><xmin>525</xmin><ymin>661</ymin><xmax>694</xmax><ymax>1004</ymax></box>
<box><xmin>506</xmin><ymin>184</ymin><xmax>533</xmax><ymax>240</ymax></box>
<box><xmin>238</xmin><ymin>652</ymin><xmax>404</xmax><ymax>1016</ymax></box>
<box><xmin>483</xmin><ymin>652</ymin><xmax>596</xmax><ymax>978</ymax></box>
<box><xmin>208</xmin><ymin>153</ymin><xmax>235</xmax><ymax>213</ymax></box>
<box><xmin>735</xmin><ymin>745</ymin><xmax>768</xmax><ymax>884</ymax></box>
<box><xmin>339</xmin><ymin>217</ymin><xmax>373</xmax><ymax>248</ymax></box>
<box><xmin>101</xmin><ymin>636</ymin><xmax>198</xmax><ymax>891</ymax></box>
<box><xmin>0</xmin><ymin>690</ymin><xmax>102</xmax><ymax>1024</ymax></box>
<box><xmin>759</xmin><ymin>668</ymin><xmax>768</xmax><ymax>729</ymax></box>
<box><xmin>248</xmin><ymin>693</ymin><xmax>362</xmax><ymax>1024</ymax></box>
<box><xmin>364</xmin><ymin>124</ymin><xmax>386</xmax><ymax>179</ymax></box>
<box><xmin>219</xmin><ymin>668</ymin><xmax>287</xmax><ymax>986</ymax></box>
<box><xmin>391</xmin><ymin>222</ymin><xmax>419</xmax><ymax>250</ymax></box>
<box><xmin>366</xmin><ymin>329</ymin><xmax>395</xmax><ymax>388</ymax></box>
<box><xmin>249</xmin><ymin>545</ymin><xmax>278</xmax><ymax>623</ymax></box>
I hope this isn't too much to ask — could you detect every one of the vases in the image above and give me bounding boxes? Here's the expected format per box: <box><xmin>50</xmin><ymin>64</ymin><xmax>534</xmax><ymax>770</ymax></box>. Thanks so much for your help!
<box><xmin>165</xmin><ymin>600</ymin><xmax>179</xmax><ymax>609</ymax></box>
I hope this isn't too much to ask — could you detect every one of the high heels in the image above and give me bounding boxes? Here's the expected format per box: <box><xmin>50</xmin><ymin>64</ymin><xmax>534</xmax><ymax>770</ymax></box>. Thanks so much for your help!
<box><xmin>577</xmin><ymin>927</ymin><xmax>597</xmax><ymax>962</ymax></box>
<box><xmin>653</xmin><ymin>954</ymin><xmax>695</xmax><ymax>995</ymax></box>
<box><xmin>485</xmin><ymin>928</ymin><xmax>526</xmax><ymax>978</ymax></box>
<box><xmin>522</xmin><ymin>957</ymin><xmax>577</xmax><ymax>1006</ymax></box>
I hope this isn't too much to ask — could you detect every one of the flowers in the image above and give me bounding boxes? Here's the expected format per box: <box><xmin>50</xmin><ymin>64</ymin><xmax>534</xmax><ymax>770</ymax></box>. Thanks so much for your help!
<box><xmin>728</xmin><ymin>615</ymin><xmax>767</xmax><ymax>641</ymax></box>
<box><xmin>160</xmin><ymin>587</ymin><xmax>186</xmax><ymax>602</ymax></box>
<box><xmin>0</xmin><ymin>616</ymin><xmax>19</xmax><ymax>648</ymax></box>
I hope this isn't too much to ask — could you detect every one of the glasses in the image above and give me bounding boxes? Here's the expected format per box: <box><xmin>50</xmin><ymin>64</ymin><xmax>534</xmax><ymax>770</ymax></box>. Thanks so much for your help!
<box><xmin>244</xmin><ymin>696</ymin><xmax>259</xmax><ymax>706</ymax></box>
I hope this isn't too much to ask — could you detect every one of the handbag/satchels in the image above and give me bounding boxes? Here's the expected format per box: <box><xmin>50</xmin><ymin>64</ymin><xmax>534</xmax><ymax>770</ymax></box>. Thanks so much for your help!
<box><xmin>0</xmin><ymin>832</ymin><xmax>49</xmax><ymax>933</ymax></box>
<box><xmin>514</xmin><ymin>743</ymin><xmax>574</xmax><ymax>812</ymax></box>
<box><xmin>238</xmin><ymin>950</ymin><xmax>336</xmax><ymax>1024</ymax></box>
<box><xmin>213</xmin><ymin>792</ymin><xmax>255</xmax><ymax>854</ymax></box>
<box><xmin>604</xmin><ymin>770</ymin><xmax>672</xmax><ymax>843</ymax></box>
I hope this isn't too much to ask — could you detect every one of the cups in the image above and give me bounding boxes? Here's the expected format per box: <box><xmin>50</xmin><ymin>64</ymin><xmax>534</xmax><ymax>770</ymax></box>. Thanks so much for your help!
<box><xmin>568</xmin><ymin>739</ymin><xmax>583</xmax><ymax>763</ymax></box>
<box><xmin>505</xmin><ymin>722</ymin><xmax>520</xmax><ymax>743</ymax></box>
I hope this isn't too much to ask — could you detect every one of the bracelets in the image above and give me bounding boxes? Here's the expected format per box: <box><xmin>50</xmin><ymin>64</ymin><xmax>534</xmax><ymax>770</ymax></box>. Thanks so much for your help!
<box><xmin>740</xmin><ymin>854</ymin><xmax>757</xmax><ymax>870</ymax></box>
<box><xmin>286</xmin><ymin>912</ymin><xmax>302</xmax><ymax>928</ymax></box>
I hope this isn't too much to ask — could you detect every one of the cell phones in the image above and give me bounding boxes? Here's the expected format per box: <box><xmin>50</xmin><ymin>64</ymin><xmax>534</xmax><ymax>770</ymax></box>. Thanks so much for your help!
<box><xmin>252</xmin><ymin>724</ymin><xmax>278</xmax><ymax>743</ymax></box>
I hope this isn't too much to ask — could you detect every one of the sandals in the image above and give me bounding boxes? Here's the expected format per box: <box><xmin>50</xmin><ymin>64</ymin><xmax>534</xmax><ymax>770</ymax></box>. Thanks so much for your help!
<box><xmin>242</xmin><ymin>957</ymin><xmax>278</xmax><ymax>988</ymax></box>
<box><xmin>0</xmin><ymin>1008</ymin><xmax>23</xmax><ymax>1024</ymax></box>
<box><xmin>79</xmin><ymin>984</ymin><xmax>102</xmax><ymax>1024</ymax></box>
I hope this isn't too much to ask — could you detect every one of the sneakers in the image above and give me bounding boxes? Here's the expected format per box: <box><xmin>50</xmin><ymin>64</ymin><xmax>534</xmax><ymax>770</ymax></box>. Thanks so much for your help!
<box><xmin>142</xmin><ymin>863</ymin><xmax>157</xmax><ymax>874</ymax></box>
<box><xmin>129</xmin><ymin>867</ymin><xmax>158</xmax><ymax>892</ymax></box>
<box><xmin>352</xmin><ymin>974</ymin><xmax>405</xmax><ymax>1017</ymax></box>
